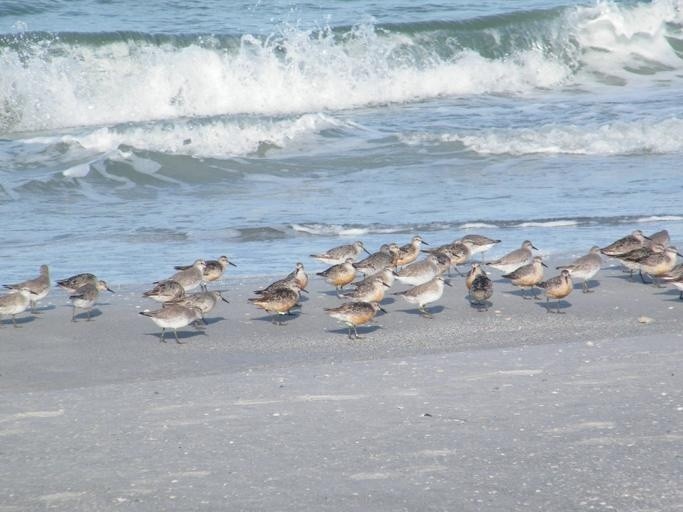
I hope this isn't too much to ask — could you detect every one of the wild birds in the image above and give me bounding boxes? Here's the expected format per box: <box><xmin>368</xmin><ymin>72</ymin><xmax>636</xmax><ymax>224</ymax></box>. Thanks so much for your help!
<box><xmin>313</xmin><ymin>233</ymin><xmax>504</xmax><ymax>339</ymax></box>
<box><xmin>54</xmin><ymin>272</ymin><xmax>99</xmax><ymax>295</ymax></box>
<box><xmin>139</xmin><ymin>254</ymin><xmax>239</xmax><ymax>345</ymax></box>
<box><xmin>0</xmin><ymin>287</ymin><xmax>38</xmax><ymax>329</ymax></box>
<box><xmin>247</xmin><ymin>262</ymin><xmax>312</xmax><ymax>326</ymax></box>
<box><xmin>2</xmin><ymin>264</ymin><xmax>50</xmax><ymax>314</ymax></box>
<box><xmin>68</xmin><ymin>280</ymin><xmax>114</xmax><ymax>322</ymax></box>
<box><xmin>488</xmin><ymin>229</ymin><xmax>683</xmax><ymax>315</ymax></box>
